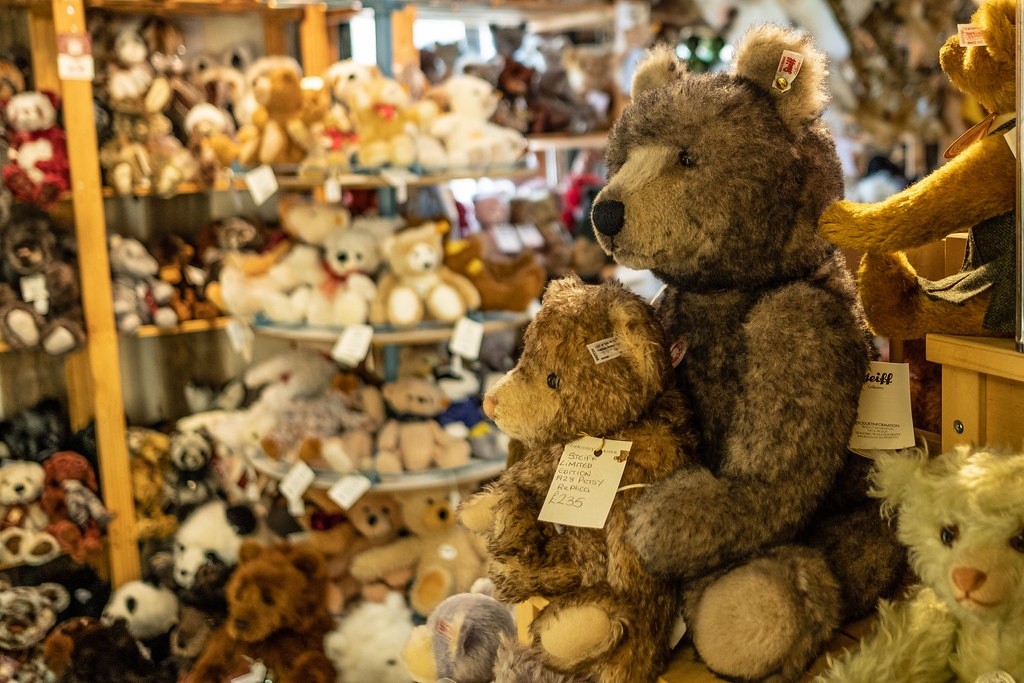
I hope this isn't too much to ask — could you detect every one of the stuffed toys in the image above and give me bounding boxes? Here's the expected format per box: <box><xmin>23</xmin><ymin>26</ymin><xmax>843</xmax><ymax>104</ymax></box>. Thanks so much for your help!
<box><xmin>815</xmin><ymin>0</ymin><xmax>1018</xmax><ymax>337</ymax></box>
<box><xmin>809</xmin><ymin>443</ymin><xmax>1024</xmax><ymax>683</ymax></box>
<box><xmin>619</xmin><ymin>0</ymin><xmax>981</xmax><ymax>204</ymax></box>
<box><xmin>0</xmin><ymin>16</ymin><xmax>668</xmax><ymax>355</ymax></box>
<box><xmin>588</xmin><ymin>23</ymin><xmax>908</xmax><ymax>683</ymax></box>
<box><xmin>456</xmin><ymin>272</ymin><xmax>701</xmax><ymax>683</ymax></box>
<box><xmin>0</xmin><ymin>327</ymin><xmax>525</xmax><ymax>683</ymax></box>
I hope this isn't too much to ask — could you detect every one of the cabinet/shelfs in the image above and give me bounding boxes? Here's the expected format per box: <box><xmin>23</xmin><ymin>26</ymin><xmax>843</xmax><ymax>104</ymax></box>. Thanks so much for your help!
<box><xmin>0</xmin><ymin>0</ymin><xmax>1023</xmax><ymax>682</ymax></box>
